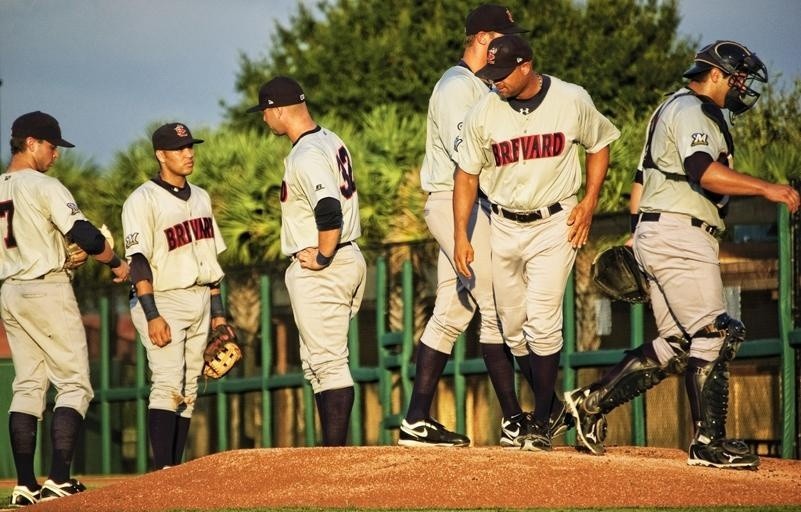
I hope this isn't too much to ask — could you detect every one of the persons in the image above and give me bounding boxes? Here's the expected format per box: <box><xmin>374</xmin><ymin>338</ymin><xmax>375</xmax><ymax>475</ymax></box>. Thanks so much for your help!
<box><xmin>0</xmin><ymin>109</ymin><xmax>131</xmax><ymax>508</ymax></box>
<box><xmin>121</xmin><ymin>122</ymin><xmax>243</xmax><ymax>469</ymax></box>
<box><xmin>397</xmin><ymin>4</ymin><xmax>536</xmax><ymax>448</ymax></box>
<box><xmin>247</xmin><ymin>75</ymin><xmax>368</xmax><ymax>446</ymax></box>
<box><xmin>452</xmin><ymin>36</ymin><xmax>622</xmax><ymax>449</ymax></box>
<box><xmin>564</xmin><ymin>41</ymin><xmax>800</xmax><ymax>469</ymax></box>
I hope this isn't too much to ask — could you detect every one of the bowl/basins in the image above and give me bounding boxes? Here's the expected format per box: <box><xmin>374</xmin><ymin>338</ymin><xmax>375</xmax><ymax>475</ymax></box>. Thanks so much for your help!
<box><xmin>535</xmin><ymin>71</ymin><xmax>546</xmax><ymax>91</ymax></box>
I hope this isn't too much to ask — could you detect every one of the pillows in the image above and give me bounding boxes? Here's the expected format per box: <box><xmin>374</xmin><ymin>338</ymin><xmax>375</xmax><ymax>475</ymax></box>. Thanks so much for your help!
<box><xmin>683</xmin><ymin>41</ymin><xmax>768</xmax><ymax>126</ymax></box>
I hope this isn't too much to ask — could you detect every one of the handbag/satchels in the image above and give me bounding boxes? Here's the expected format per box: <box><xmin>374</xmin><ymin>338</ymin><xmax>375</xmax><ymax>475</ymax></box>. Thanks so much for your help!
<box><xmin>137</xmin><ymin>292</ymin><xmax>161</xmax><ymax>320</ymax></box>
<box><xmin>316</xmin><ymin>250</ymin><xmax>334</xmax><ymax>268</ymax></box>
<box><xmin>211</xmin><ymin>293</ymin><xmax>227</xmax><ymax>318</ymax></box>
<box><xmin>629</xmin><ymin>212</ymin><xmax>641</xmax><ymax>232</ymax></box>
<box><xmin>108</xmin><ymin>253</ymin><xmax>123</xmax><ymax>270</ymax></box>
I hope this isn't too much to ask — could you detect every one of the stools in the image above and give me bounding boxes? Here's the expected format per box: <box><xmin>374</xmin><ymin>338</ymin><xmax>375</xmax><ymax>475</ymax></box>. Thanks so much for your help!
<box><xmin>642</xmin><ymin>213</ymin><xmax>721</xmax><ymax>238</ymax></box>
<box><xmin>288</xmin><ymin>241</ymin><xmax>353</xmax><ymax>262</ymax></box>
<box><xmin>490</xmin><ymin>202</ymin><xmax>564</xmax><ymax>224</ymax></box>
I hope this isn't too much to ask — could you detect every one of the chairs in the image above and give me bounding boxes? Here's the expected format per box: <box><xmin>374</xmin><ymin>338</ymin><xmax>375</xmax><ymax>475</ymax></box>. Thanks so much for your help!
<box><xmin>686</xmin><ymin>438</ymin><xmax>760</xmax><ymax>468</ymax></box>
<box><xmin>499</xmin><ymin>412</ymin><xmax>522</xmax><ymax>447</ymax></box>
<box><xmin>8</xmin><ymin>485</ymin><xmax>41</xmax><ymax>508</ymax></box>
<box><xmin>564</xmin><ymin>386</ymin><xmax>609</xmax><ymax>454</ymax></box>
<box><xmin>518</xmin><ymin>419</ymin><xmax>554</xmax><ymax>452</ymax></box>
<box><xmin>514</xmin><ymin>401</ymin><xmax>578</xmax><ymax>446</ymax></box>
<box><xmin>397</xmin><ymin>415</ymin><xmax>471</xmax><ymax>449</ymax></box>
<box><xmin>41</xmin><ymin>479</ymin><xmax>88</xmax><ymax>503</ymax></box>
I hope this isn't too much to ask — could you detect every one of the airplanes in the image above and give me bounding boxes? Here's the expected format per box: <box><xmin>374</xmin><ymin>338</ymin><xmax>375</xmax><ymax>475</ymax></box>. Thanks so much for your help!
<box><xmin>202</xmin><ymin>324</ymin><xmax>243</xmax><ymax>380</ymax></box>
<box><xmin>592</xmin><ymin>246</ymin><xmax>651</xmax><ymax>304</ymax></box>
<box><xmin>63</xmin><ymin>239</ymin><xmax>88</xmax><ymax>270</ymax></box>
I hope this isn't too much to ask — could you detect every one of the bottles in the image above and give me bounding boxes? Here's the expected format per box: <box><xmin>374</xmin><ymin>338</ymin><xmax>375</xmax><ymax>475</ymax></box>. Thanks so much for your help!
<box><xmin>152</xmin><ymin>124</ymin><xmax>206</xmax><ymax>151</ymax></box>
<box><xmin>474</xmin><ymin>36</ymin><xmax>530</xmax><ymax>82</ymax></box>
<box><xmin>466</xmin><ymin>6</ymin><xmax>531</xmax><ymax>36</ymax></box>
<box><xmin>247</xmin><ymin>77</ymin><xmax>306</xmax><ymax>113</ymax></box>
<box><xmin>11</xmin><ymin>111</ymin><xmax>76</xmax><ymax>148</ymax></box>
